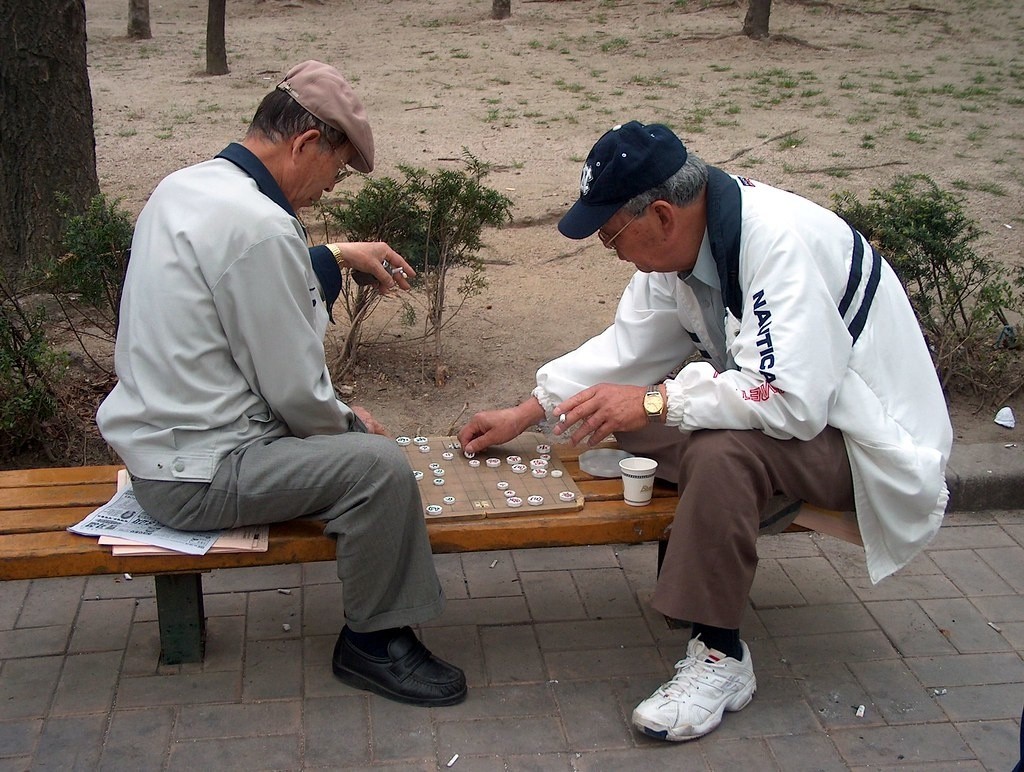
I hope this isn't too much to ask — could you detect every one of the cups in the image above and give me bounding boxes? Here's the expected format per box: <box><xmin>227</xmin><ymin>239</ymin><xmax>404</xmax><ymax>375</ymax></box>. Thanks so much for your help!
<box><xmin>619</xmin><ymin>457</ymin><xmax>658</xmax><ymax>506</ymax></box>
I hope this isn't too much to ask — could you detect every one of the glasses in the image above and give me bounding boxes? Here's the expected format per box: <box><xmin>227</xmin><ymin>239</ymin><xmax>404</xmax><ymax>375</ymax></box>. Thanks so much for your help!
<box><xmin>311</xmin><ymin>128</ymin><xmax>352</xmax><ymax>183</ymax></box>
<box><xmin>598</xmin><ymin>199</ymin><xmax>665</xmax><ymax>249</ymax></box>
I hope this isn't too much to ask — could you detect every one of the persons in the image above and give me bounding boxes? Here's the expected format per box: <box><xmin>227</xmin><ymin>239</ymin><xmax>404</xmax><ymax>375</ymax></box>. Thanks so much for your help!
<box><xmin>95</xmin><ymin>61</ymin><xmax>466</xmax><ymax>708</ymax></box>
<box><xmin>458</xmin><ymin>122</ymin><xmax>953</xmax><ymax>741</ymax></box>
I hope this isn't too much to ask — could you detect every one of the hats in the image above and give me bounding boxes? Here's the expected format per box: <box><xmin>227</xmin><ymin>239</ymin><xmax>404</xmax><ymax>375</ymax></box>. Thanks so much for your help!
<box><xmin>557</xmin><ymin>120</ymin><xmax>687</xmax><ymax>240</ymax></box>
<box><xmin>276</xmin><ymin>59</ymin><xmax>375</xmax><ymax>173</ymax></box>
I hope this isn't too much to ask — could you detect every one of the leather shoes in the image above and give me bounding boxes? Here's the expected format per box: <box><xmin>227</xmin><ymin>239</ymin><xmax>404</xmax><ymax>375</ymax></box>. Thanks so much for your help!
<box><xmin>330</xmin><ymin>625</ymin><xmax>467</xmax><ymax>707</ymax></box>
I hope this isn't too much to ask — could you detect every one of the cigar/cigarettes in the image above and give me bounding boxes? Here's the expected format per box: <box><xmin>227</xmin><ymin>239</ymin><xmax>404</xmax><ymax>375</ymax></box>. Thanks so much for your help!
<box><xmin>559</xmin><ymin>413</ymin><xmax>566</xmax><ymax>423</ymax></box>
<box><xmin>400</xmin><ymin>270</ymin><xmax>409</xmax><ymax>279</ymax></box>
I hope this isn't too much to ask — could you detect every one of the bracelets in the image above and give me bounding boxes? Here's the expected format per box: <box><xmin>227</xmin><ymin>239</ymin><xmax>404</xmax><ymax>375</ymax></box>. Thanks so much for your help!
<box><xmin>325</xmin><ymin>244</ymin><xmax>344</xmax><ymax>270</ymax></box>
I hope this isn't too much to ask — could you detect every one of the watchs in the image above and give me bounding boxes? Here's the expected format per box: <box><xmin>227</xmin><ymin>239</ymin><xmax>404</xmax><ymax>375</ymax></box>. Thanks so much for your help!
<box><xmin>643</xmin><ymin>385</ymin><xmax>664</xmax><ymax>428</ymax></box>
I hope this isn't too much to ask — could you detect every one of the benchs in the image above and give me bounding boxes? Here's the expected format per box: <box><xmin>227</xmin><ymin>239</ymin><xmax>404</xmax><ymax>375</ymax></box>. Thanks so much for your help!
<box><xmin>0</xmin><ymin>440</ymin><xmax>817</xmax><ymax>665</ymax></box>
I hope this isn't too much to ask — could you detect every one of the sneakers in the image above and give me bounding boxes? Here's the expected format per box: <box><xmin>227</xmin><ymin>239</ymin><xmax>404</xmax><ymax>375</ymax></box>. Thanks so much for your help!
<box><xmin>631</xmin><ymin>633</ymin><xmax>757</xmax><ymax>741</ymax></box>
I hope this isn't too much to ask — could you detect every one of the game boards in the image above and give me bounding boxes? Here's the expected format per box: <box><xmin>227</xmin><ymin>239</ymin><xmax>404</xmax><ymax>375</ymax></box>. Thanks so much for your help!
<box><xmin>391</xmin><ymin>431</ymin><xmax>587</xmax><ymax>526</ymax></box>
